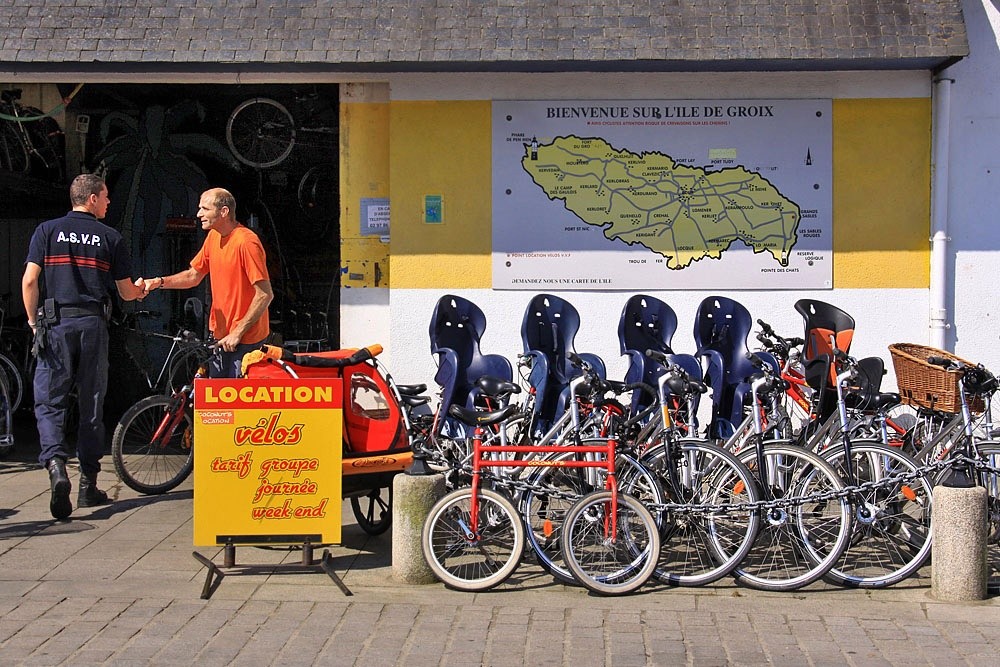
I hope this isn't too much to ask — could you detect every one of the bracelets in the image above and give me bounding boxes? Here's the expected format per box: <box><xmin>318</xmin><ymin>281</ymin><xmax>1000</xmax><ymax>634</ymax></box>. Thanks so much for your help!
<box><xmin>155</xmin><ymin>276</ymin><xmax>164</xmax><ymax>289</ymax></box>
<box><xmin>28</xmin><ymin>320</ymin><xmax>37</xmax><ymax>329</ymax></box>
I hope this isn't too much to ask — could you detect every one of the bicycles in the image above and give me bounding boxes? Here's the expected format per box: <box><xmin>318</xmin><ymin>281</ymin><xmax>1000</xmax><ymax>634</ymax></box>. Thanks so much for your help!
<box><xmin>350</xmin><ymin>318</ymin><xmax>1000</xmax><ymax>598</ymax></box>
<box><xmin>107</xmin><ymin>310</ymin><xmax>220</xmax><ymax>495</ymax></box>
<box><xmin>0</xmin><ymin>308</ymin><xmax>25</xmax><ymax>459</ymax></box>
<box><xmin>225</xmin><ymin>84</ymin><xmax>340</xmax><ymax>222</ymax></box>
<box><xmin>0</xmin><ymin>89</ymin><xmax>64</xmax><ymax>197</ymax></box>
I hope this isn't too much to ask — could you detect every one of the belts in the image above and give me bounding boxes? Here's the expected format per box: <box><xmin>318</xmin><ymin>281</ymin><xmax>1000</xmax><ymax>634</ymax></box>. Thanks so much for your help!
<box><xmin>56</xmin><ymin>308</ymin><xmax>97</xmax><ymax>318</ymax></box>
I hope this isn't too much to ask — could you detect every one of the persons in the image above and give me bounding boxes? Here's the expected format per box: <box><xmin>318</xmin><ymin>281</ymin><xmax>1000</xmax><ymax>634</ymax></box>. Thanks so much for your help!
<box><xmin>21</xmin><ymin>174</ymin><xmax>144</xmax><ymax>520</ymax></box>
<box><xmin>137</xmin><ymin>188</ymin><xmax>275</xmax><ymax>378</ymax></box>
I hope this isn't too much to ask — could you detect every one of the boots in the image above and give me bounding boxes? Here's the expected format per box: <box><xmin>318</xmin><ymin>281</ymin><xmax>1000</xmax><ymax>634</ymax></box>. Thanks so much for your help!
<box><xmin>47</xmin><ymin>458</ymin><xmax>74</xmax><ymax>518</ymax></box>
<box><xmin>77</xmin><ymin>473</ymin><xmax>108</xmax><ymax>507</ymax></box>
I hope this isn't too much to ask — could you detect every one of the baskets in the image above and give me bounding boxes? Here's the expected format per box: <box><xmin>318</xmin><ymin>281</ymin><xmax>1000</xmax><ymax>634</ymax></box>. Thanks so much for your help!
<box><xmin>888</xmin><ymin>343</ymin><xmax>984</xmax><ymax>413</ymax></box>
